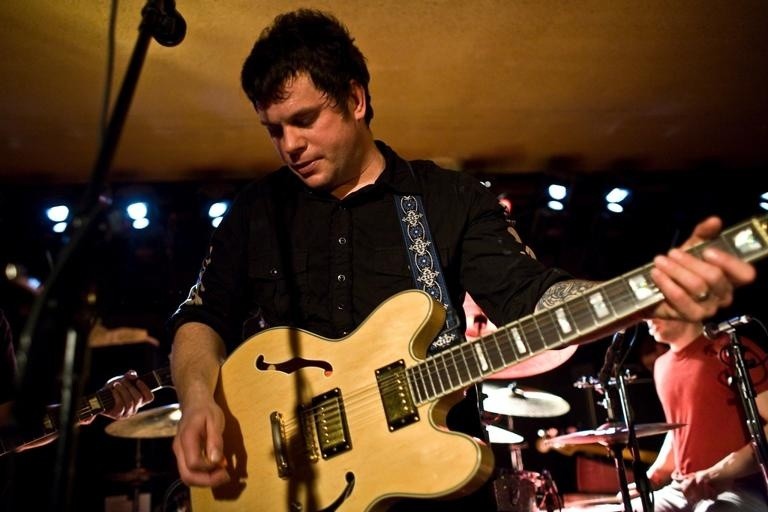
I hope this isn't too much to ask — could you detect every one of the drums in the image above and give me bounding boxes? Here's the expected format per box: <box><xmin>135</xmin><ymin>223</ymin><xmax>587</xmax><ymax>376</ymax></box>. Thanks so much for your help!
<box><xmin>496</xmin><ymin>470</ymin><xmax>565</xmax><ymax>511</ymax></box>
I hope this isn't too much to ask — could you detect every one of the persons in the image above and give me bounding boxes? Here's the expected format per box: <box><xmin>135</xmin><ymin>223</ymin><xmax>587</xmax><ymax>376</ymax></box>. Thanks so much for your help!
<box><xmin>599</xmin><ymin>312</ymin><xmax>767</xmax><ymax>511</ymax></box>
<box><xmin>1</xmin><ymin>313</ymin><xmax>156</xmax><ymax>454</ymax></box>
<box><xmin>169</xmin><ymin>9</ymin><xmax>756</xmax><ymax>511</ymax></box>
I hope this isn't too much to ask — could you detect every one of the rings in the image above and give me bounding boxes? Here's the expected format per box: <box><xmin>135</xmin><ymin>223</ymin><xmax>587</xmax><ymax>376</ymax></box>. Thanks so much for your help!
<box><xmin>696</xmin><ymin>290</ymin><xmax>710</xmax><ymax>301</ymax></box>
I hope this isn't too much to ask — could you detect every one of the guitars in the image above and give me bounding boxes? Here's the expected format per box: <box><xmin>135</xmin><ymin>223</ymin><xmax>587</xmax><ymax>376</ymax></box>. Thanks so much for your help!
<box><xmin>0</xmin><ymin>366</ymin><xmax>173</xmax><ymax>458</ymax></box>
<box><xmin>188</xmin><ymin>214</ymin><xmax>768</xmax><ymax>512</ymax></box>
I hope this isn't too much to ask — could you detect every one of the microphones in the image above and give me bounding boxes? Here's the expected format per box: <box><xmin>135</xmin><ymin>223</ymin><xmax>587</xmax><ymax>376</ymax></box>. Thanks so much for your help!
<box><xmin>145</xmin><ymin>0</ymin><xmax>187</xmax><ymax>48</ymax></box>
<box><xmin>701</xmin><ymin>314</ymin><xmax>753</xmax><ymax>342</ymax></box>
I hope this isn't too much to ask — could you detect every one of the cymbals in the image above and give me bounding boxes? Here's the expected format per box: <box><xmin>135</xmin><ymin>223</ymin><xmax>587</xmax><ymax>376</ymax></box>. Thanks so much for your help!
<box><xmin>482</xmin><ymin>383</ymin><xmax>571</xmax><ymax>418</ymax></box>
<box><xmin>486</xmin><ymin>422</ymin><xmax>523</xmax><ymax>447</ymax></box>
<box><xmin>545</xmin><ymin>423</ymin><xmax>687</xmax><ymax>448</ymax></box>
<box><xmin>105</xmin><ymin>401</ymin><xmax>181</xmax><ymax>438</ymax></box>
